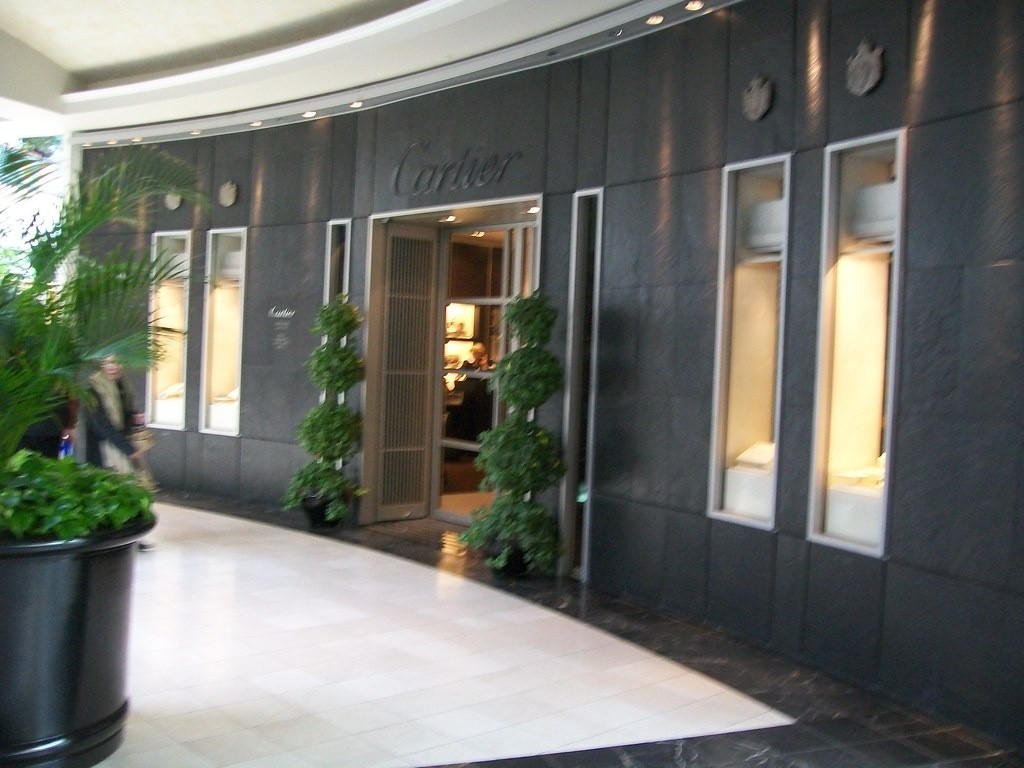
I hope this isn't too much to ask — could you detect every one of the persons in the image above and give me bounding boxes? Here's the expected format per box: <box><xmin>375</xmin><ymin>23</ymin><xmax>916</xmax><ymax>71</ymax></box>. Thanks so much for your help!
<box><xmin>446</xmin><ymin>343</ymin><xmax>499</xmax><ymax>463</ymax></box>
<box><xmin>84</xmin><ymin>352</ymin><xmax>160</xmax><ymax>551</ymax></box>
<box><xmin>18</xmin><ymin>387</ymin><xmax>79</xmax><ymax>460</ymax></box>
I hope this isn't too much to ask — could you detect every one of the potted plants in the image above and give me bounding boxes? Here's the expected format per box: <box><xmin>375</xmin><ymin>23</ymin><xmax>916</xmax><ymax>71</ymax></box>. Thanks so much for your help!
<box><xmin>0</xmin><ymin>141</ymin><xmax>213</xmax><ymax>768</ymax></box>
<box><xmin>279</xmin><ymin>296</ymin><xmax>371</xmax><ymax>528</ymax></box>
<box><xmin>457</xmin><ymin>287</ymin><xmax>563</xmax><ymax>580</ymax></box>
